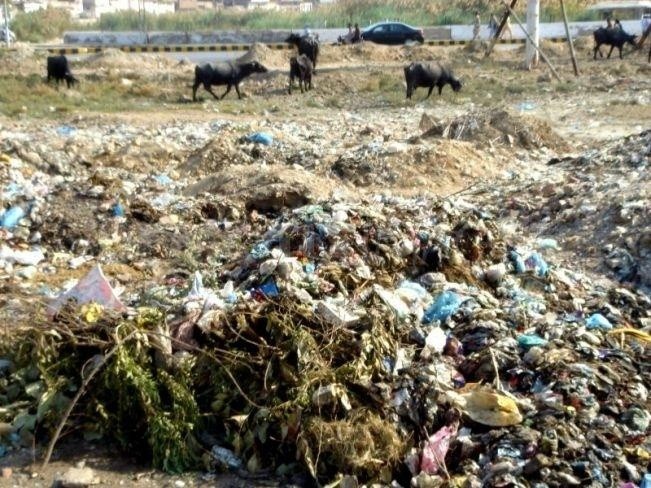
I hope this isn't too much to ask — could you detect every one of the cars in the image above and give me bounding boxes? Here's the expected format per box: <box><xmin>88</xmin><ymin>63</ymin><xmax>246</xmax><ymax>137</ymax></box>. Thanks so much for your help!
<box><xmin>0</xmin><ymin>28</ymin><xmax>17</xmax><ymax>44</ymax></box>
<box><xmin>357</xmin><ymin>21</ymin><xmax>425</xmax><ymax>47</ymax></box>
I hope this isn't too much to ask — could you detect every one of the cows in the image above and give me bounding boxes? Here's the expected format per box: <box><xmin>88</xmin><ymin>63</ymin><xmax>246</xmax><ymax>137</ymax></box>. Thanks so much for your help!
<box><xmin>192</xmin><ymin>60</ymin><xmax>267</xmax><ymax>103</ymax></box>
<box><xmin>47</xmin><ymin>55</ymin><xmax>81</xmax><ymax>90</ymax></box>
<box><xmin>404</xmin><ymin>60</ymin><xmax>463</xmax><ymax>100</ymax></box>
<box><xmin>288</xmin><ymin>53</ymin><xmax>313</xmax><ymax>94</ymax></box>
<box><xmin>592</xmin><ymin>27</ymin><xmax>638</xmax><ymax>61</ymax></box>
<box><xmin>283</xmin><ymin>32</ymin><xmax>320</xmax><ymax>75</ymax></box>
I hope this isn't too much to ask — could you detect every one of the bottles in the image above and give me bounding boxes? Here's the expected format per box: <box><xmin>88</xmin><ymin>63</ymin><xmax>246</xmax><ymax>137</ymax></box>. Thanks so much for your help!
<box><xmin>213</xmin><ymin>445</ymin><xmax>239</xmax><ymax>467</ymax></box>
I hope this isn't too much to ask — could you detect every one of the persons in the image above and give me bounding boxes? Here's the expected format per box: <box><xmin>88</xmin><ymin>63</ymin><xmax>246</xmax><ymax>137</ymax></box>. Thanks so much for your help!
<box><xmin>614</xmin><ymin>19</ymin><xmax>623</xmax><ymax>30</ymax></box>
<box><xmin>502</xmin><ymin>14</ymin><xmax>514</xmax><ymax>42</ymax></box>
<box><xmin>351</xmin><ymin>22</ymin><xmax>362</xmax><ymax>45</ymax></box>
<box><xmin>606</xmin><ymin>17</ymin><xmax>612</xmax><ymax>30</ymax></box>
<box><xmin>469</xmin><ymin>9</ymin><xmax>482</xmax><ymax>41</ymax></box>
<box><xmin>345</xmin><ymin>22</ymin><xmax>353</xmax><ymax>40</ymax></box>
<box><xmin>488</xmin><ymin>12</ymin><xmax>501</xmax><ymax>39</ymax></box>
